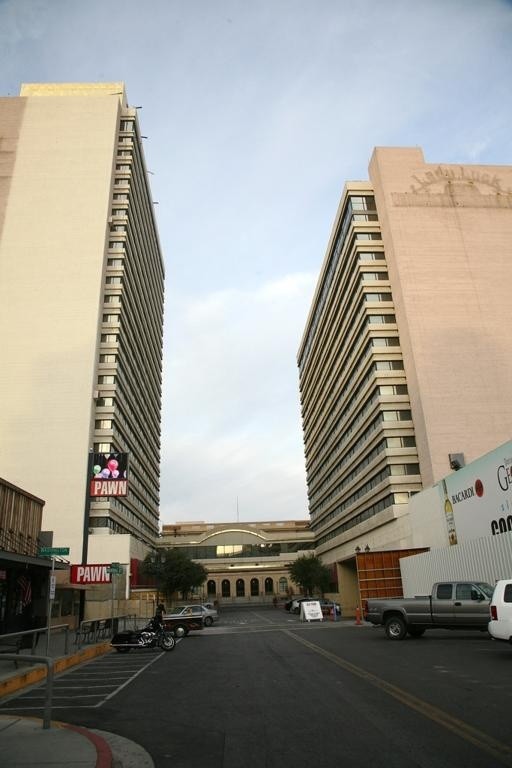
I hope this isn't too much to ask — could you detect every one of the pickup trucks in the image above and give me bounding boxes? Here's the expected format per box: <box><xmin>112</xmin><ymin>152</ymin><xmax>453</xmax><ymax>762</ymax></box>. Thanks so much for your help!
<box><xmin>363</xmin><ymin>580</ymin><xmax>492</xmax><ymax>641</ymax></box>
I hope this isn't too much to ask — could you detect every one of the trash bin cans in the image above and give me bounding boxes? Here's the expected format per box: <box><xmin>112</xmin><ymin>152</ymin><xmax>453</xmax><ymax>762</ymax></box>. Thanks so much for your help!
<box><xmin>106</xmin><ymin>618</ymin><xmax>118</xmax><ymax>635</ymax></box>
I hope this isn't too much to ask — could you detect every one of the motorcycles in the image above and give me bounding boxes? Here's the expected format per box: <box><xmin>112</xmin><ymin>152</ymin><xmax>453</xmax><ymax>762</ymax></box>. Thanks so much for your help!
<box><xmin>111</xmin><ymin>621</ymin><xmax>177</xmax><ymax>653</ymax></box>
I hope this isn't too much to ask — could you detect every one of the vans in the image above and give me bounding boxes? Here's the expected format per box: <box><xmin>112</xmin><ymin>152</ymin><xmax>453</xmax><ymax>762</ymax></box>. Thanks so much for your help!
<box><xmin>488</xmin><ymin>580</ymin><xmax>511</xmax><ymax>641</ymax></box>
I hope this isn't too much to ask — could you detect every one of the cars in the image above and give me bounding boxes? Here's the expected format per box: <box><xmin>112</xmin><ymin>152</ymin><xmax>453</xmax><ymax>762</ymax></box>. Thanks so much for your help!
<box><xmin>285</xmin><ymin>596</ymin><xmax>340</xmax><ymax>615</ymax></box>
<box><xmin>148</xmin><ymin>599</ymin><xmax>220</xmax><ymax>636</ymax></box>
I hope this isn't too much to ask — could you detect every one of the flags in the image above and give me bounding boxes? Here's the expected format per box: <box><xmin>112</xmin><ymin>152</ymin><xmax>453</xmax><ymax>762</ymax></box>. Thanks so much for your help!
<box><xmin>17</xmin><ymin>568</ymin><xmax>31</xmax><ymax>607</ymax></box>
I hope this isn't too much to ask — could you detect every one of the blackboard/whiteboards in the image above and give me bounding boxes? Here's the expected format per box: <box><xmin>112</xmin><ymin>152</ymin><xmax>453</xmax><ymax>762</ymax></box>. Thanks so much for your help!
<box><xmin>301</xmin><ymin>600</ymin><xmax>324</xmax><ymax>620</ymax></box>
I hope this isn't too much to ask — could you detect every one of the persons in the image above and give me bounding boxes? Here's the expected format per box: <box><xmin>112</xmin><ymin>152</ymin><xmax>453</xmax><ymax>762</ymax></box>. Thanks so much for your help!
<box><xmin>155</xmin><ymin>598</ymin><xmax>166</xmax><ymax>625</ymax></box>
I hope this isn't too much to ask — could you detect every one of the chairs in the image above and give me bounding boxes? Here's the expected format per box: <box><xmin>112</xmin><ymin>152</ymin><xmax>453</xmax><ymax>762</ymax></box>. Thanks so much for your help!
<box><xmin>1</xmin><ymin>626</ymin><xmax>44</xmax><ymax>664</ymax></box>
<box><xmin>74</xmin><ymin>618</ymin><xmax>118</xmax><ymax>644</ymax></box>
